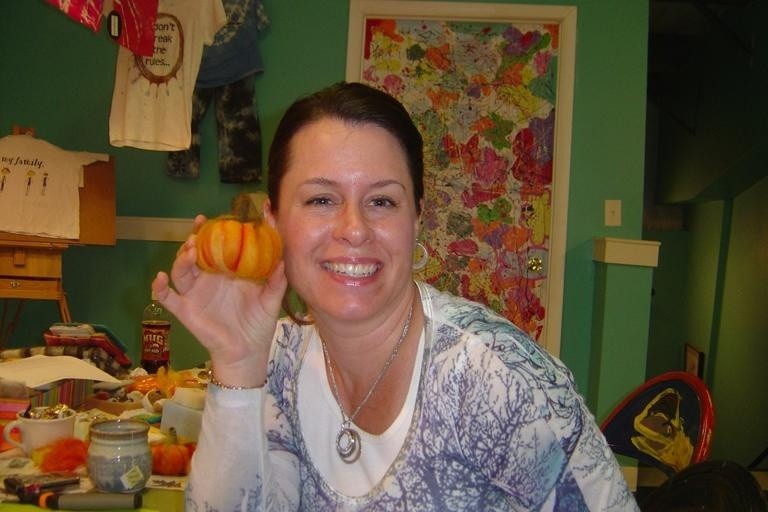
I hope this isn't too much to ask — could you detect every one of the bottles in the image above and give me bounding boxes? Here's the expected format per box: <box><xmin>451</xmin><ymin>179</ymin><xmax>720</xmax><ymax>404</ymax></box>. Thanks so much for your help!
<box><xmin>143</xmin><ymin>300</ymin><xmax>170</xmax><ymax>373</ymax></box>
<box><xmin>86</xmin><ymin>421</ymin><xmax>150</xmax><ymax>495</ymax></box>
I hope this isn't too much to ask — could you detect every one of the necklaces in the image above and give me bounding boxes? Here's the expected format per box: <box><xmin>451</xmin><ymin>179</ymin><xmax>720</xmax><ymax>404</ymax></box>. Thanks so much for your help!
<box><xmin>323</xmin><ymin>297</ymin><xmax>413</xmax><ymax>465</ymax></box>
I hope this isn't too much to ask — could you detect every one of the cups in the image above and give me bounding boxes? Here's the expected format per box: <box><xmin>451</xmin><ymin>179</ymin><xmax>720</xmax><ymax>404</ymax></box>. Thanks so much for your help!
<box><xmin>5</xmin><ymin>405</ymin><xmax>77</xmax><ymax>457</ymax></box>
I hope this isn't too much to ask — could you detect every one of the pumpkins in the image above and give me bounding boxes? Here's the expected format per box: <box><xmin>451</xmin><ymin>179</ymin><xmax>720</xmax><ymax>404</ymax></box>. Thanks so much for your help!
<box><xmin>148</xmin><ymin>427</ymin><xmax>197</xmax><ymax>476</ymax></box>
<box><xmin>194</xmin><ymin>190</ymin><xmax>283</xmax><ymax>280</ymax></box>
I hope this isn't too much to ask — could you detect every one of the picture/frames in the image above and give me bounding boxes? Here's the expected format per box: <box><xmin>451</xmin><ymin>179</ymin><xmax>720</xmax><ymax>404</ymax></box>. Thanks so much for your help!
<box><xmin>684</xmin><ymin>343</ymin><xmax>704</xmax><ymax>380</ymax></box>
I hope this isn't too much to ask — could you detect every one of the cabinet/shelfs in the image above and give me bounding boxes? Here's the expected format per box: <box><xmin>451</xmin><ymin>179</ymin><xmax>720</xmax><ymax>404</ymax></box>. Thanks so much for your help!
<box><xmin>2</xmin><ymin>128</ymin><xmax>117</xmax><ymax>351</ymax></box>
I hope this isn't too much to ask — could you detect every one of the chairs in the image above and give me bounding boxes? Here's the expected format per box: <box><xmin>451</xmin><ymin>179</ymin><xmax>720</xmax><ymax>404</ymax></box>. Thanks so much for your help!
<box><xmin>598</xmin><ymin>371</ymin><xmax>713</xmax><ymax>474</ymax></box>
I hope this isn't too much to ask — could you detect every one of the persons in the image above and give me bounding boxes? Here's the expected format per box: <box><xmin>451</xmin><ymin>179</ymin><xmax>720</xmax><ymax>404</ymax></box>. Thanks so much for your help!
<box><xmin>150</xmin><ymin>79</ymin><xmax>641</xmax><ymax>512</ymax></box>
